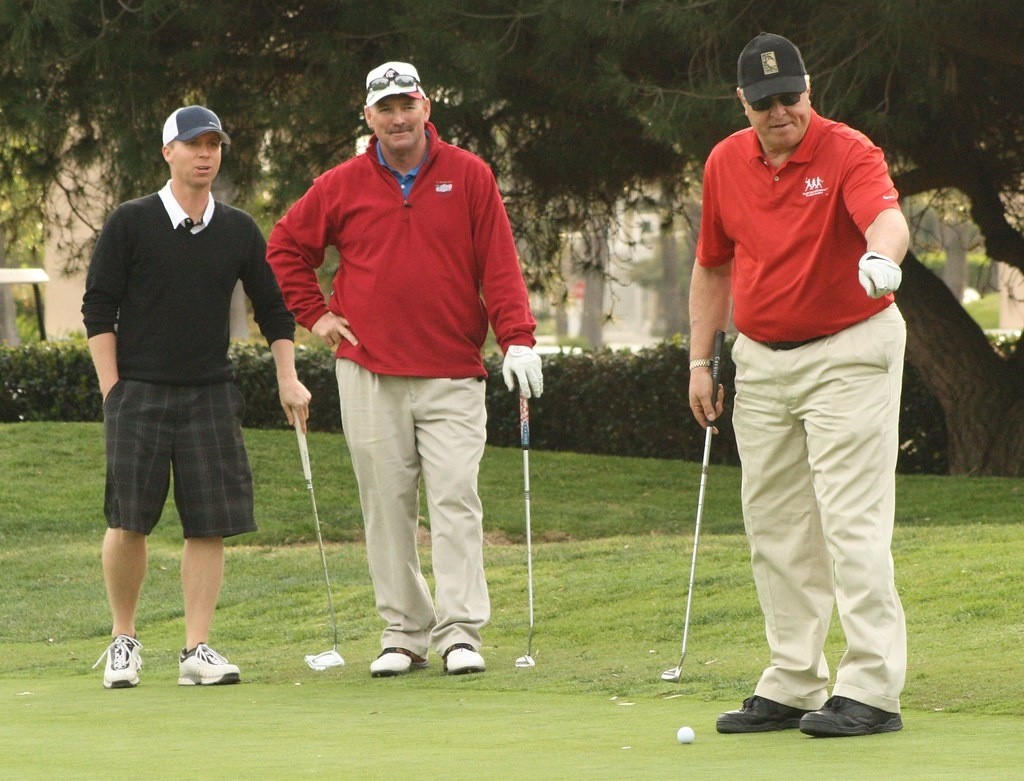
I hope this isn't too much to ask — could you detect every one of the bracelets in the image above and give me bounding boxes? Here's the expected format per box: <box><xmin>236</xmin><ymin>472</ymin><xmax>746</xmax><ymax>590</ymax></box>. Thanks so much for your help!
<box><xmin>689</xmin><ymin>359</ymin><xmax>712</xmax><ymax>369</ymax></box>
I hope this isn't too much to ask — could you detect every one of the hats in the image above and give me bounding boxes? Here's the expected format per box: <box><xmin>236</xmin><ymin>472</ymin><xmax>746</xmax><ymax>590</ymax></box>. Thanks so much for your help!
<box><xmin>365</xmin><ymin>61</ymin><xmax>426</xmax><ymax>107</ymax></box>
<box><xmin>162</xmin><ymin>105</ymin><xmax>231</xmax><ymax>146</ymax></box>
<box><xmin>738</xmin><ymin>32</ymin><xmax>808</xmax><ymax>103</ymax></box>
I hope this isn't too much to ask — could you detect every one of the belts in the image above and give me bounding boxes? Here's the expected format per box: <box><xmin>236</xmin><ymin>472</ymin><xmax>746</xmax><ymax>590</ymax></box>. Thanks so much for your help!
<box><xmin>759</xmin><ymin>336</ymin><xmax>827</xmax><ymax>351</ymax></box>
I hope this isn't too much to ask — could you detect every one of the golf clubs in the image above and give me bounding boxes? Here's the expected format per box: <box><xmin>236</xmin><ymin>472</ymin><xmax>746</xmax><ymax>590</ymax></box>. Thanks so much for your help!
<box><xmin>289</xmin><ymin>405</ymin><xmax>345</xmax><ymax>668</ymax></box>
<box><xmin>661</xmin><ymin>328</ymin><xmax>727</xmax><ymax>683</ymax></box>
<box><xmin>513</xmin><ymin>383</ymin><xmax>536</xmax><ymax>668</ymax></box>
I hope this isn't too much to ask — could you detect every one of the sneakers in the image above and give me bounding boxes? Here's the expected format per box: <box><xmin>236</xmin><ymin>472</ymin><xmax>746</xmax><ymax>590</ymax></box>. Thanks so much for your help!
<box><xmin>716</xmin><ymin>695</ymin><xmax>819</xmax><ymax>733</ymax></box>
<box><xmin>370</xmin><ymin>648</ymin><xmax>430</xmax><ymax>674</ymax></box>
<box><xmin>91</xmin><ymin>634</ymin><xmax>143</xmax><ymax>689</ymax></box>
<box><xmin>799</xmin><ymin>696</ymin><xmax>903</xmax><ymax>736</ymax></box>
<box><xmin>177</xmin><ymin>642</ymin><xmax>240</xmax><ymax>685</ymax></box>
<box><xmin>442</xmin><ymin>643</ymin><xmax>486</xmax><ymax>675</ymax></box>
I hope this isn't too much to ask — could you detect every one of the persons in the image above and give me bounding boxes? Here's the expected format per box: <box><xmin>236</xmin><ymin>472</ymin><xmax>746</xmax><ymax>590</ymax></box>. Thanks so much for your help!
<box><xmin>688</xmin><ymin>31</ymin><xmax>912</xmax><ymax>738</ymax></box>
<box><xmin>81</xmin><ymin>105</ymin><xmax>315</xmax><ymax>687</ymax></box>
<box><xmin>263</xmin><ymin>61</ymin><xmax>544</xmax><ymax>675</ymax></box>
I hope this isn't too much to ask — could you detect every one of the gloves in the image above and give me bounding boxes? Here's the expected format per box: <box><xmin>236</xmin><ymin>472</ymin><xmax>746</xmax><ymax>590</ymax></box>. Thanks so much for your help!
<box><xmin>502</xmin><ymin>344</ymin><xmax>544</xmax><ymax>398</ymax></box>
<box><xmin>858</xmin><ymin>252</ymin><xmax>902</xmax><ymax>299</ymax></box>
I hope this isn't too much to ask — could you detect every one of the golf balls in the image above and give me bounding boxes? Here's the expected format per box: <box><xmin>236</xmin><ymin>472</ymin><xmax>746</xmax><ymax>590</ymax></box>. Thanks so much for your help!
<box><xmin>677</xmin><ymin>727</ymin><xmax>695</xmax><ymax>744</ymax></box>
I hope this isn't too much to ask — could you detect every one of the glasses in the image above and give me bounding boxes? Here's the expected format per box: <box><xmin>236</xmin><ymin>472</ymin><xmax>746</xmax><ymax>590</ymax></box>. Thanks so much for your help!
<box><xmin>366</xmin><ymin>75</ymin><xmax>427</xmax><ymax>97</ymax></box>
<box><xmin>751</xmin><ymin>92</ymin><xmax>803</xmax><ymax>111</ymax></box>
